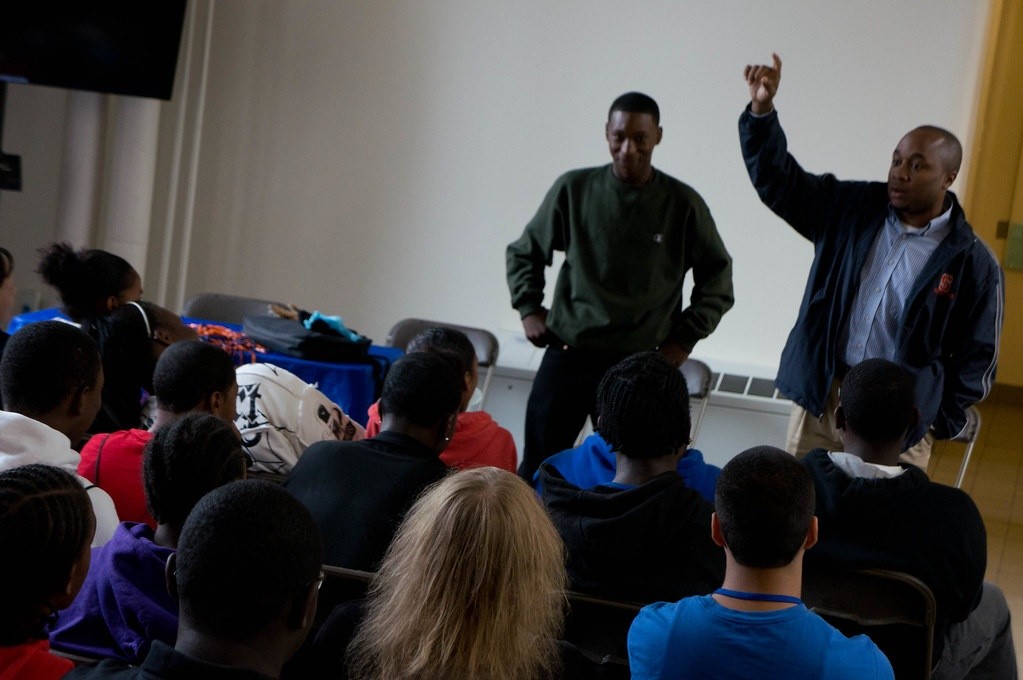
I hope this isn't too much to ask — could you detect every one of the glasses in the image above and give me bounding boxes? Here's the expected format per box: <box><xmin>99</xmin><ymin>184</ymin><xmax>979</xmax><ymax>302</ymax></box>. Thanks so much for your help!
<box><xmin>299</xmin><ymin>571</ymin><xmax>327</xmax><ymax>593</ymax></box>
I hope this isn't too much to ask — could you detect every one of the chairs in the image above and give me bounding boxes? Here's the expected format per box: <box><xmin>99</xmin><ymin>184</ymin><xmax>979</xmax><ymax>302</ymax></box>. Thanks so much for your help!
<box><xmin>185</xmin><ymin>293</ymin><xmax>287</xmax><ymax>333</ymax></box>
<box><xmin>577</xmin><ymin>355</ymin><xmax>716</xmax><ymax>453</ymax></box>
<box><xmin>383</xmin><ymin>317</ymin><xmax>501</xmax><ymax>411</ymax></box>
<box><xmin>315</xmin><ymin>561</ymin><xmax>946</xmax><ymax>680</ymax></box>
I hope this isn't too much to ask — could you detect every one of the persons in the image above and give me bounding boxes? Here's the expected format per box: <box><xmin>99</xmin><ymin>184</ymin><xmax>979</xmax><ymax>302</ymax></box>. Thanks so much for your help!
<box><xmin>505</xmin><ymin>92</ymin><xmax>734</xmax><ymax>484</ymax></box>
<box><xmin>0</xmin><ymin>242</ymin><xmax>1019</xmax><ymax>680</ymax></box>
<box><xmin>737</xmin><ymin>53</ymin><xmax>1005</xmax><ymax>473</ymax></box>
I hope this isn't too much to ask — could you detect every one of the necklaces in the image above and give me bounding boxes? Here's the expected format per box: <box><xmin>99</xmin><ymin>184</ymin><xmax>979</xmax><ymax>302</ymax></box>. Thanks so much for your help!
<box><xmin>714</xmin><ymin>589</ymin><xmax>802</xmax><ymax>605</ymax></box>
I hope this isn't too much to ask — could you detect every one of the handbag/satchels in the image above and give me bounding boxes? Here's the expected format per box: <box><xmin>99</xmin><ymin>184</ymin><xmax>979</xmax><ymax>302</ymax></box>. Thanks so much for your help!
<box><xmin>241</xmin><ymin>314</ymin><xmax>374</xmax><ymax>362</ymax></box>
